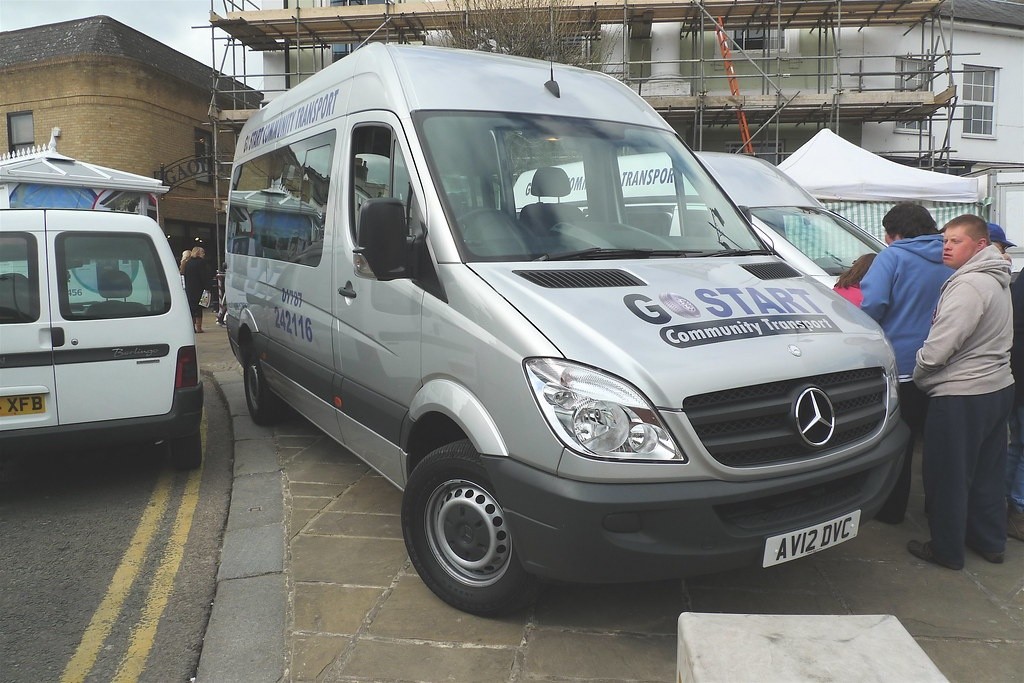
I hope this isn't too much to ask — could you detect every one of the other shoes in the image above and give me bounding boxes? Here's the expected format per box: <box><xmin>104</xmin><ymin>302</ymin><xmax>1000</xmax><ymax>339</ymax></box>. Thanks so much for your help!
<box><xmin>977</xmin><ymin>552</ymin><xmax>1004</xmax><ymax>563</ymax></box>
<box><xmin>907</xmin><ymin>539</ymin><xmax>963</xmax><ymax>570</ymax></box>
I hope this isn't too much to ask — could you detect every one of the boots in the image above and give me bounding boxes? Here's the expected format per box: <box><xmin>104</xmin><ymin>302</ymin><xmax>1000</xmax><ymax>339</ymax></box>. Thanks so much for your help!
<box><xmin>192</xmin><ymin>317</ymin><xmax>204</xmax><ymax>333</ymax></box>
<box><xmin>1005</xmin><ymin>500</ymin><xmax>1024</xmax><ymax>541</ymax></box>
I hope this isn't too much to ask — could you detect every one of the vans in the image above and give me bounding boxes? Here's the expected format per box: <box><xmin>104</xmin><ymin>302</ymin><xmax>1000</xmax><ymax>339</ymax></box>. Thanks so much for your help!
<box><xmin>513</xmin><ymin>150</ymin><xmax>889</xmax><ymax>290</ymax></box>
<box><xmin>0</xmin><ymin>208</ymin><xmax>205</xmax><ymax>468</ymax></box>
<box><xmin>224</xmin><ymin>42</ymin><xmax>911</xmax><ymax>614</ymax></box>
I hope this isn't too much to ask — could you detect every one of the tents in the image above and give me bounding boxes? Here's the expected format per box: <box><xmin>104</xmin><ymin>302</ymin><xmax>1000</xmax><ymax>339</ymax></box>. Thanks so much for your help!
<box><xmin>775</xmin><ymin>128</ymin><xmax>989</xmax><ymax>267</ymax></box>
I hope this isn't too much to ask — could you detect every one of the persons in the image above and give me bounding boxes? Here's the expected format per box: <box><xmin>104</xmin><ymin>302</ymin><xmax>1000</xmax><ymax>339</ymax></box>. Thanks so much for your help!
<box><xmin>180</xmin><ymin>247</ymin><xmax>207</xmax><ymax>333</ymax></box>
<box><xmin>833</xmin><ymin>202</ymin><xmax>1024</xmax><ymax>570</ymax></box>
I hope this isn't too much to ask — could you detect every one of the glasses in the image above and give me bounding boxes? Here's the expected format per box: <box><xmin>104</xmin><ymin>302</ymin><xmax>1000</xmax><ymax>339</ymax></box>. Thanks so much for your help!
<box><xmin>1000</xmin><ymin>244</ymin><xmax>1008</xmax><ymax>250</ymax></box>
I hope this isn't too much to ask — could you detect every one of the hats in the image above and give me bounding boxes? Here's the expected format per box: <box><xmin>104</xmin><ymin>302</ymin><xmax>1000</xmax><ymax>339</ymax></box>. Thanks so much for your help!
<box><xmin>986</xmin><ymin>223</ymin><xmax>1017</xmax><ymax>247</ymax></box>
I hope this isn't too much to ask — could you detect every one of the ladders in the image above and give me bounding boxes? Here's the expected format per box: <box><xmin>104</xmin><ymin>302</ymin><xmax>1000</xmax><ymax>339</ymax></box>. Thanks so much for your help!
<box><xmin>714</xmin><ymin>18</ymin><xmax>754</xmax><ymax>153</ymax></box>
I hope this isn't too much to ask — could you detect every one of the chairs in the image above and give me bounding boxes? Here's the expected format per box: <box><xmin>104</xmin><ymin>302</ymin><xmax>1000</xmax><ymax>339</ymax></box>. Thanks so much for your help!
<box><xmin>82</xmin><ymin>270</ymin><xmax>150</xmax><ymax>319</ymax></box>
<box><xmin>0</xmin><ymin>272</ymin><xmax>30</xmax><ymax>324</ymax></box>
<box><xmin>519</xmin><ymin>167</ymin><xmax>592</xmax><ymax>261</ymax></box>
